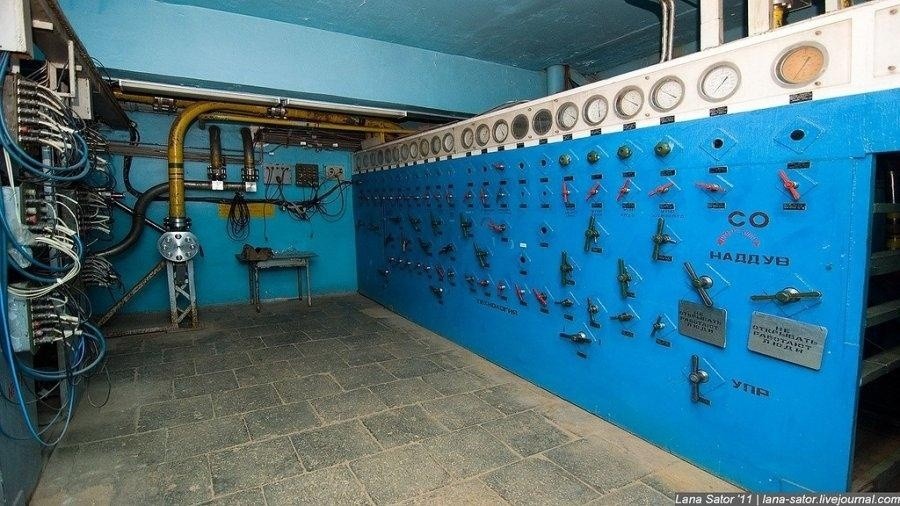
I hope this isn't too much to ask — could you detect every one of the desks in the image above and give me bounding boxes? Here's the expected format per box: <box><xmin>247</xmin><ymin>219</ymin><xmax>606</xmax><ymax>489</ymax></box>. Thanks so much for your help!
<box><xmin>235</xmin><ymin>248</ymin><xmax>319</xmax><ymax>312</ymax></box>
